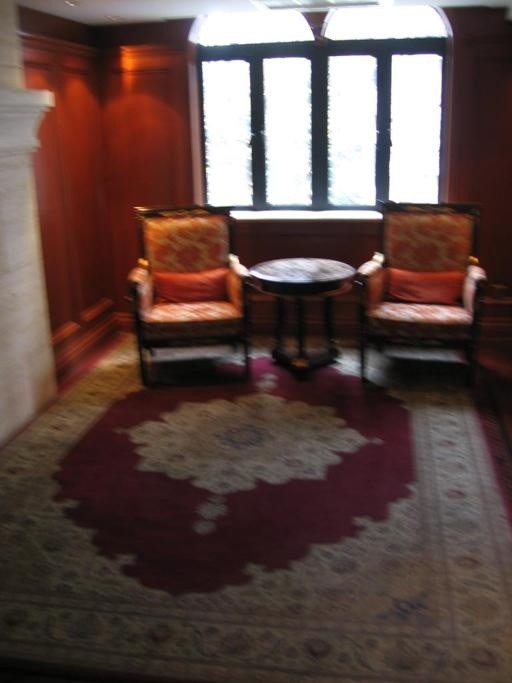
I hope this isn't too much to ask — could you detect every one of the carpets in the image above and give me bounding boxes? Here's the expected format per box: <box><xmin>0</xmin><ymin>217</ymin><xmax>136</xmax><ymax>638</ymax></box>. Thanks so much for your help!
<box><xmin>1</xmin><ymin>324</ymin><xmax>510</xmax><ymax>679</ymax></box>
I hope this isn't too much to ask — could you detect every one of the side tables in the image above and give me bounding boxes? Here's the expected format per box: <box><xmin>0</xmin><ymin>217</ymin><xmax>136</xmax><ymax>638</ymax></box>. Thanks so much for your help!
<box><xmin>248</xmin><ymin>258</ymin><xmax>357</xmax><ymax>374</ymax></box>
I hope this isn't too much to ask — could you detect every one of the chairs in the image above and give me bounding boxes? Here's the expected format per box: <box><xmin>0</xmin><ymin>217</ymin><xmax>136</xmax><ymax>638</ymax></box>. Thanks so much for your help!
<box><xmin>358</xmin><ymin>194</ymin><xmax>497</xmax><ymax>408</ymax></box>
<box><xmin>129</xmin><ymin>206</ymin><xmax>253</xmax><ymax>390</ymax></box>
